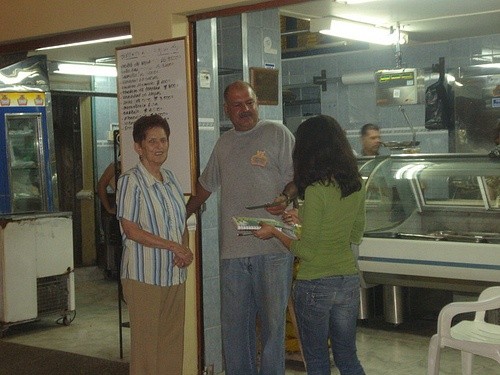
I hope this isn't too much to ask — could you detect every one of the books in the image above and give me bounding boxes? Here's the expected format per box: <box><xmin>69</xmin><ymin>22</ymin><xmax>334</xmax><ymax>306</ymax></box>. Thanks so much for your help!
<box><xmin>231</xmin><ymin>216</ymin><xmax>283</xmax><ymax>231</ymax></box>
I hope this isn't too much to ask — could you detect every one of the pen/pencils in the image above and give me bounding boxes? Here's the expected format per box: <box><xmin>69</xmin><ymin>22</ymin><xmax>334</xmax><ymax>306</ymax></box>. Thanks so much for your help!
<box><xmin>237</xmin><ymin>233</ymin><xmax>254</xmax><ymax>236</ymax></box>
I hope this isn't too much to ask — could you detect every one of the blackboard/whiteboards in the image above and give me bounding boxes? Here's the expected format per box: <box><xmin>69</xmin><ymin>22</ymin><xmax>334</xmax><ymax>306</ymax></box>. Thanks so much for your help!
<box><xmin>115</xmin><ymin>36</ymin><xmax>196</xmax><ymax>196</ymax></box>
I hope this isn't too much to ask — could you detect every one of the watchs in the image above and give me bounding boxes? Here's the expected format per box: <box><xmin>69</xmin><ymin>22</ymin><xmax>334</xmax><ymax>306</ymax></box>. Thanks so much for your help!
<box><xmin>283</xmin><ymin>193</ymin><xmax>290</xmax><ymax>206</ymax></box>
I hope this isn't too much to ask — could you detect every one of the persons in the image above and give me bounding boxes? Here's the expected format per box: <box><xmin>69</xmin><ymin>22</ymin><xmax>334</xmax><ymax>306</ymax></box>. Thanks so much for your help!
<box><xmin>97</xmin><ymin>156</ymin><xmax>121</xmax><ymax>281</ymax></box>
<box><xmin>359</xmin><ymin>124</ymin><xmax>381</xmax><ymax>157</ymax></box>
<box><xmin>186</xmin><ymin>81</ymin><xmax>298</xmax><ymax>375</ymax></box>
<box><xmin>250</xmin><ymin>115</ymin><xmax>367</xmax><ymax>375</ymax></box>
<box><xmin>115</xmin><ymin>114</ymin><xmax>193</xmax><ymax>375</ymax></box>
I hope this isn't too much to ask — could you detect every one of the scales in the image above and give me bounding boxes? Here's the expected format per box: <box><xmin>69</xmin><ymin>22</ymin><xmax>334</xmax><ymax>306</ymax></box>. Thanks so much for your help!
<box><xmin>379</xmin><ymin>141</ymin><xmax>420</xmax><ymax>153</ymax></box>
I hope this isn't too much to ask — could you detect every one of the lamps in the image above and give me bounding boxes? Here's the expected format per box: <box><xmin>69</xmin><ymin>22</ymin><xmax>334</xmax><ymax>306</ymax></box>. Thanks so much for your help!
<box><xmin>310</xmin><ymin>17</ymin><xmax>408</xmax><ymax>45</ymax></box>
<box><xmin>48</xmin><ymin>62</ymin><xmax>117</xmax><ymax>76</ymax></box>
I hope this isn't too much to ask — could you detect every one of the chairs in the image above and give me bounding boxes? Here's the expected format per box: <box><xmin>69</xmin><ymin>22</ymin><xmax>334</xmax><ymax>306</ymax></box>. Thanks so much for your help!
<box><xmin>427</xmin><ymin>286</ymin><xmax>500</xmax><ymax>375</ymax></box>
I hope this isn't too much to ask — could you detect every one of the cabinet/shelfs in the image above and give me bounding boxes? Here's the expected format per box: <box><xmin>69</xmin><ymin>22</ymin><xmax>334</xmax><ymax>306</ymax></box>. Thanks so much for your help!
<box><xmin>282</xmin><ymin>83</ymin><xmax>338</xmax><ymax>133</ymax></box>
